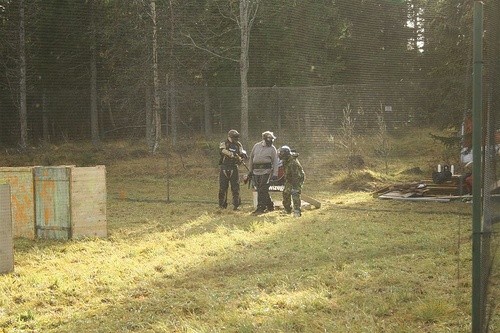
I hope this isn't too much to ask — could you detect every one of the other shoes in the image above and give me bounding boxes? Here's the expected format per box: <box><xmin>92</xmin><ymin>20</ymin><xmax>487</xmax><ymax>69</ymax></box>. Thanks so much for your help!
<box><xmin>220</xmin><ymin>202</ymin><xmax>227</xmax><ymax>209</ymax></box>
<box><xmin>266</xmin><ymin>206</ymin><xmax>274</xmax><ymax>212</ymax></box>
<box><xmin>252</xmin><ymin>205</ymin><xmax>264</xmax><ymax>213</ymax></box>
<box><xmin>282</xmin><ymin>209</ymin><xmax>292</xmax><ymax>215</ymax></box>
<box><xmin>233</xmin><ymin>207</ymin><xmax>240</xmax><ymax>212</ymax></box>
<box><xmin>294</xmin><ymin>208</ymin><xmax>301</xmax><ymax>216</ymax></box>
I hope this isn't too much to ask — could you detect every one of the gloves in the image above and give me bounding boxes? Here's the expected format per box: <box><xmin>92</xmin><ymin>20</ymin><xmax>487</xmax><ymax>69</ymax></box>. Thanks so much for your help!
<box><xmin>234</xmin><ymin>156</ymin><xmax>242</xmax><ymax>165</ymax></box>
<box><xmin>241</xmin><ymin>151</ymin><xmax>248</xmax><ymax>159</ymax></box>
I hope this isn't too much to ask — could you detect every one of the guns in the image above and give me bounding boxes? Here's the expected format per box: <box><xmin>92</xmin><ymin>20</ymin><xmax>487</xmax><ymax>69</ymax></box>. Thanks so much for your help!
<box><xmin>234</xmin><ymin>153</ymin><xmax>250</xmax><ymax>172</ymax></box>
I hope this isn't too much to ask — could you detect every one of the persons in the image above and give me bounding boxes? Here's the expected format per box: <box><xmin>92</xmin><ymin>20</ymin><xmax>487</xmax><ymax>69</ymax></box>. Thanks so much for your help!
<box><xmin>249</xmin><ymin>131</ymin><xmax>279</xmax><ymax>214</ymax></box>
<box><xmin>279</xmin><ymin>146</ymin><xmax>305</xmax><ymax>218</ymax></box>
<box><xmin>219</xmin><ymin>129</ymin><xmax>247</xmax><ymax>212</ymax></box>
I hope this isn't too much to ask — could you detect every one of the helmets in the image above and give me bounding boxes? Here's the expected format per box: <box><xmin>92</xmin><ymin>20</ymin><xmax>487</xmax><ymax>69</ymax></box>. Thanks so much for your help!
<box><xmin>277</xmin><ymin>145</ymin><xmax>291</xmax><ymax>159</ymax></box>
<box><xmin>262</xmin><ymin>131</ymin><xmax>276</xmax><ymax>141</ymax></box>
<box><xmin>228</xmin><ymin>130</ymin><xmax>240</xmax><ymax>139</ymax></box>
